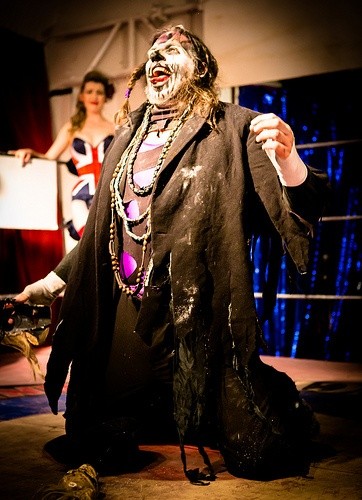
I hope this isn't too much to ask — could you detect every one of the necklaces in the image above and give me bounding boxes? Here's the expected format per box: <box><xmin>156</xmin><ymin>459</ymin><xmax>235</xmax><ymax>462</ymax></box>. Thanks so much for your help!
<box><xmin>108</xmin><ymin>99</ymin><xmax>195</xmax><ymax>297</ymax></box>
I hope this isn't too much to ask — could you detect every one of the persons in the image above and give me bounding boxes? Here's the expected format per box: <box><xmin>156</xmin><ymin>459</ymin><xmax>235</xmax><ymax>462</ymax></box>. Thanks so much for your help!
<box><xmin>0</xmin><ymin>23</ymin><xmax>338</xmax><ymax>483</ymax></box>
<box><xmin>6</xmin><ymin>70</ymin><xmax>120</xmax><ymax>254</ymax></box>
